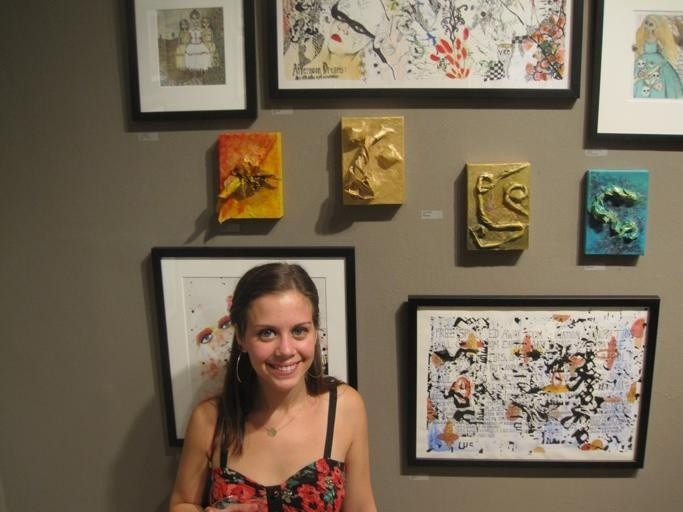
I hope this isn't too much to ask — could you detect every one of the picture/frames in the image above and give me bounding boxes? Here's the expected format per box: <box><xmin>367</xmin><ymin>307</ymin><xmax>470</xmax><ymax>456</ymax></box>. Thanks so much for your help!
<box><xmin>126</xmin><ymin>0</ymin><xmax>261</xmax><ymax>121</ymax></box>
<box><xmin>261</xmin><ymin>0</ymin><xmax>585</xmax><ymax>99</ymax></box>
<box><xmin>579</xmin><ymin>0</ymin><xmax>682</xmax><ymax>147</ymax></box>
<box><xmin>149</xmin><ymin>243</ymin><xmax>358</xmax><ymax>447</ymax></box>
<box><xmin>405</xmin><ymin>295</ymin><xmax>660</xmax><ymax>467</ymax></box>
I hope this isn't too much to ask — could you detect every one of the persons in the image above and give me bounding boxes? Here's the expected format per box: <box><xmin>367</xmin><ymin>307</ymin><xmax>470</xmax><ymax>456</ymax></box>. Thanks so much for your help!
<box><xmin>174</xmin><ymin>9</ymin><xmax>222</xmax><ymax>84</ymax></box>
<box><xmin>634</xmin><ymin>14</ymin><xmax>683</xmax><ymax>98</ymax></box>
<box><xmin>283</xmin><ymin>0</ymin><xmax>385</xmax><ymax>81</ymax></box>
<box><xmin>168</xmin><ymin>263</ymin><xmax>381</xmax><ymax>512</ymax></box>
